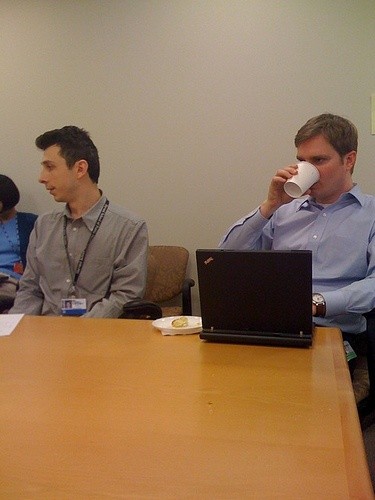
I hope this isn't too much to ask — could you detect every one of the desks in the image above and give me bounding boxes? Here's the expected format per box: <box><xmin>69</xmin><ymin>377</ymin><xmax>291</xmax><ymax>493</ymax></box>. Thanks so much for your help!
<box><xmin>0</xmin><ymin>315</ymin><xmax>375</xmax><ymax>500</ymax></box>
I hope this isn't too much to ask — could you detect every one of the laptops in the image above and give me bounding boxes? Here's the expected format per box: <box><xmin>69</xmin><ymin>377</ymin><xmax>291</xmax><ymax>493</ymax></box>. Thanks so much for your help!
<box><xmin>196</xmin><ymin>249</ymin><xmax>315</xmax><ymax>346</ymax></box>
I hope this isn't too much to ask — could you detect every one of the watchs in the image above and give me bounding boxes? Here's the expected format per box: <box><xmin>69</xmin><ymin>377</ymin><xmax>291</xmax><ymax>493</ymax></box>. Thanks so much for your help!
<box><xmin>312</xmin><ymin>294</ymin><xmax>326</xmax><ymax>318</ymax></box>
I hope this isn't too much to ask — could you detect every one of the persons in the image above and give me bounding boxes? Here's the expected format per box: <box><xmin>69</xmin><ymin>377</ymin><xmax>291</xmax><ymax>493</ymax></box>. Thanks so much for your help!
<box><xmin>218</xmin><ymin>114</ymin><xmax>375</xmax><ymax>380</ymax></box>
<box><xmin>0</xmin><ymin>174</ymin><xmax>40</xmax><ymax>314</ymax></box>
<box><xmin>8</xmin><ymin>126</ymin><xmax>148</xmax><ymax>318</ymax></box>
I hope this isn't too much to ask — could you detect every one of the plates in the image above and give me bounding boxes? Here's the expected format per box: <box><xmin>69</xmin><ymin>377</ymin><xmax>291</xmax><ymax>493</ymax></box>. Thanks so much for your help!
<box><xmin>152</xmin><ymin>316</ymin><xmax>202</xmax><ymax>334</ymax></box>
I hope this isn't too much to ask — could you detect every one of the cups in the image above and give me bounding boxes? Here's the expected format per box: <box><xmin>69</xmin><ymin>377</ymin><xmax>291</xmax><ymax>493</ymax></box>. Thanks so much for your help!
<box><xmin>283</xmin><ymin>161</ymin><xmax>320</xmax><ymax>198</ymax></box>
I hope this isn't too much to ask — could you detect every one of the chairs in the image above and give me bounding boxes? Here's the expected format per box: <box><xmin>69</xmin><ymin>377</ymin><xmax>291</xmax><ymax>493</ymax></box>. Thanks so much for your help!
<box><xmin>340</xmin><ymin>310</ymin><xmax>375</xmax><ymax>404</ymax></box>
<box><xmin>118</xmin><ymin>246</ymin><xmax>194</xmax><ymax>320</ymax></box>
